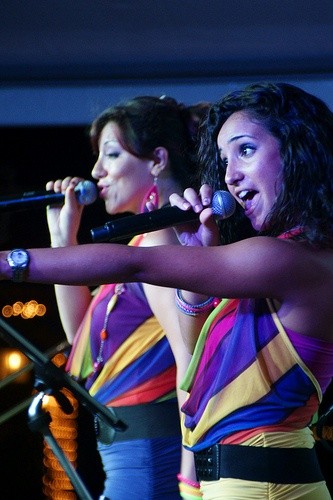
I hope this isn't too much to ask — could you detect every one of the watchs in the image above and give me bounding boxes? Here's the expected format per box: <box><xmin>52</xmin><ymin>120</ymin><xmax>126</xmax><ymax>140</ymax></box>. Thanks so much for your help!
<box><xmin>4</xmin><ymin>247</ymin><xmax>32</xmax><ymax>287</ymax></box>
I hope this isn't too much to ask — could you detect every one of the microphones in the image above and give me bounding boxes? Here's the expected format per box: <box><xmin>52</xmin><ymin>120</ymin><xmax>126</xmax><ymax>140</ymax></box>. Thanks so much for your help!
<box><xmin>90</xmin><ymin>190</ymin><xmax>236</xmax><ymax>243</ymax></box>
<box><xmin>0</xmin><ymin>180</ymin><xmax>96</xmax><ymax>212</ymax></box>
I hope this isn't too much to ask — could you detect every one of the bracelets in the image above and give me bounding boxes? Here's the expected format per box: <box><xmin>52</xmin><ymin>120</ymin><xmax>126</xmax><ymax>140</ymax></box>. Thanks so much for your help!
<box><xmin>173</xmin><ymin>287</ymin><xmax>215</xmax><ymax>317</ymax></box>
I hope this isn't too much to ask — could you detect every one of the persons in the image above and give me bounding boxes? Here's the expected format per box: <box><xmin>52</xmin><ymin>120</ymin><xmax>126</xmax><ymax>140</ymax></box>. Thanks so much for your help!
<box><xmin>44</xmin><ymin>93</ymin><xmax>219</xmax><ymax>499</ymax></box>
<box><xmin>0</xmin><ymin>79</ymin><xmax>333</xmax><ymax>500</ymax></box>
